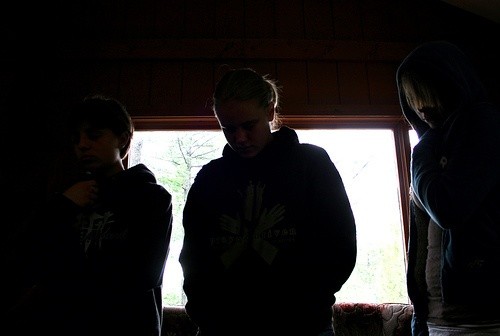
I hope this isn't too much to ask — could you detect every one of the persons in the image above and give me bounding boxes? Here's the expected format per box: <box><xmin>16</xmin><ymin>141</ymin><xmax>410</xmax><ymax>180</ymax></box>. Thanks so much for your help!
<box><xmin>395</xmin><ymin>39</ymin><xmax>500</xmax><ymax>336</ymax></box>
<box><xmin>177</xmin><ymin>63</ymin><xmax>357</xmax><ymax>336</ymax></box>
<box><xmin>24</xmin><ymin>92</ymin><xmax>173</xmax><ymax>336</ymax></box>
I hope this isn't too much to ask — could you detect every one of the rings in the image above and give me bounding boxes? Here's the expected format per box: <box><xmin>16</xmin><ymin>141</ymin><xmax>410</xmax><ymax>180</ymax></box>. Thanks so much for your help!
<box><xmin>91</xmin><ymin>186</ymin><xmax>95</xmax><ymax>191</ymax></box>
